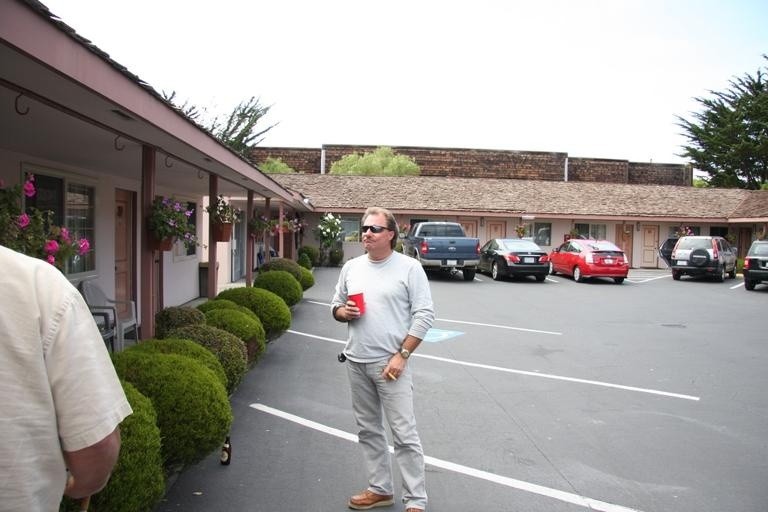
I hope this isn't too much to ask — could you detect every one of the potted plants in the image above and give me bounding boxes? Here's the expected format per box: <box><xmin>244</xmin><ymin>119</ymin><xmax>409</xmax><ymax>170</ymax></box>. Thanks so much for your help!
<box><xmin>205</xmin><ymin>194</ymin><xmax>242</xmax><ymax>241</ymax></box>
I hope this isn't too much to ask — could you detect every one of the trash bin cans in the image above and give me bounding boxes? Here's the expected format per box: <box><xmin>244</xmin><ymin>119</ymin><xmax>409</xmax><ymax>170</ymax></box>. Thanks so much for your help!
<box><xmin>199</xmin><ymin>262</ymin><xmax>219</xmax><ymax>297</ymax></box>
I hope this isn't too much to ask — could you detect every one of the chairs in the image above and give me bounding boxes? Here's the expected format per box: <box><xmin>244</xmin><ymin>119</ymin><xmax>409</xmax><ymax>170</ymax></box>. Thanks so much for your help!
<box><xmin>78</xmin><ymin>279</ymin><xmax>138</xmax><ymax>353</ymax></box>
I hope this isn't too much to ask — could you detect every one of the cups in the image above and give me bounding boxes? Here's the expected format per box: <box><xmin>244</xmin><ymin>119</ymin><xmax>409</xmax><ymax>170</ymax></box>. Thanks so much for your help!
<box><xmin>347</xmin><ymin>291</ymin><xmax>364</xmax><ymax>313</ymax></box>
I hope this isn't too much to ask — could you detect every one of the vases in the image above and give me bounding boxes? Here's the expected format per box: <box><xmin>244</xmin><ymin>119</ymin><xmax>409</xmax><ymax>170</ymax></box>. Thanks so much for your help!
<box><xmin>159</xmin><ymin>237</ymin><xmax>172</xmax><ymax>250</ymax></box>
<box><xmin>256</xmin><ymin>233</ymin><xmax>263</xmax><ymax>241</ymax></box>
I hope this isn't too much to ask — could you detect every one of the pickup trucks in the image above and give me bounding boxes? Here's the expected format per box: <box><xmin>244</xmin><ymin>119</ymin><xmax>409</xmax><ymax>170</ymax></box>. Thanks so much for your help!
<box><xmin>400</xmin><ymin>222</ymin><xmax>481</xmax><ymax>281</ymax></box>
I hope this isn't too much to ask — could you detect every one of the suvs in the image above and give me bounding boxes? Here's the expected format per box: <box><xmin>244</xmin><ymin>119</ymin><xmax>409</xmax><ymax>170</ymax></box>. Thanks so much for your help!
<box><xmin>658</xmin><ymin>236</ymin><xmax>768</xmax><ymax>291</ymax></box>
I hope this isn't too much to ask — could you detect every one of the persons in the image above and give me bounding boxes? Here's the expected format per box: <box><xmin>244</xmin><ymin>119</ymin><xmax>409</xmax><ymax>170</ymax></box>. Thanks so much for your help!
<box><xmin>327</xmin><ymin>204</ymin><xmax>438</xmax><ymax>512</ymax></box>
<box><xmin>0</xmin><ymin>244</ymin><xmax>136</xmax><ymax>511</ymax></box>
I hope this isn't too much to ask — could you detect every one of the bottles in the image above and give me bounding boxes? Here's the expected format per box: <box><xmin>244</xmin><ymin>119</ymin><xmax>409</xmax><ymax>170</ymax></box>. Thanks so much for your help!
<box><xmin>221</xmin><ymin>435</ymin><xmax>232</xmax><ymax>465</ymax></box>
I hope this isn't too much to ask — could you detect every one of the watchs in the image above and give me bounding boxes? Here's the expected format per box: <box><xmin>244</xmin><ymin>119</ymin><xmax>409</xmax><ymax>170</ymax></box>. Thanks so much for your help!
<box><xmin>398</xmin><ymin>346</ymin><xmax>411</xmax><ymax>359</ymax></box>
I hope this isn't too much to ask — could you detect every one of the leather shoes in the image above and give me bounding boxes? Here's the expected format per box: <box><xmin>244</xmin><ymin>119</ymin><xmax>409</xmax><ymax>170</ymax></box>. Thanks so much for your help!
<box><xmin>348</xmin><ymin>491</ymin><xmax>395</xmax><ymax>509</ymax></box>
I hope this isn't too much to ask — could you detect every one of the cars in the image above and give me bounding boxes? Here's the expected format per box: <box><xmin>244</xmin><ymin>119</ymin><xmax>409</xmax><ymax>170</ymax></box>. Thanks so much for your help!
<box><xmin>475</xmin><ymin>239</ymin><xmax>628</xmax><ymax>283</ymax></box>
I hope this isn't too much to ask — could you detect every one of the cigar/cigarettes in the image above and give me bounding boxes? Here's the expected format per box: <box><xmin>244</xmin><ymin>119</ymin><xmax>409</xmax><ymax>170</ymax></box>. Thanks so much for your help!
<box><xmin>382</xmin><ymin>367</ymin><xmax>397</xmax><ymax>382</ymax></box>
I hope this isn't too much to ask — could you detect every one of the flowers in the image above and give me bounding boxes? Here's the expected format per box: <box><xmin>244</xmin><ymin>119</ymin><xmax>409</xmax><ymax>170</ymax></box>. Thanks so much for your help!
<box><xmin>249</xmin><ymin>215</ymin><xmax>302</xmax><ymax>235</ymax></box>
<box><xmin>148</xmin><ymin>192</ymin><xmax>208</xmax><ymax>250</ymax></box>
<box><xmin>674</xmin><ymin>224</ymin><xmax>694</xmax><ymax>237</ymax></box>
<box><xmin>512</xmin><ymin>222</ymin><xmax>527</xmax><ymax>238</ymax></box>
<box><xmin>1</xmin><ymin>171</ymin><xmax>91</xmax><ymax>274</ymax></box>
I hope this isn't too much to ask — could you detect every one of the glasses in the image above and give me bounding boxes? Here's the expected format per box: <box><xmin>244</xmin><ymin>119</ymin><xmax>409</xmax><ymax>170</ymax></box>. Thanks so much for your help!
<box><xmin>362</xmin><ymin>226</ymin><xmax>392</xmax><ymax>232</ymax></box>
